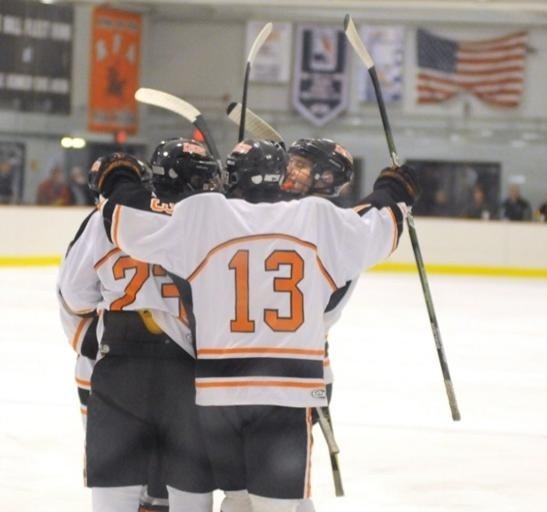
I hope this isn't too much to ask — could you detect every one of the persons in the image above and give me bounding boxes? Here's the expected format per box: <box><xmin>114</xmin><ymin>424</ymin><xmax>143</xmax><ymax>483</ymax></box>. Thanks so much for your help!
<box><xmin>503</xmin><ymin>182</ymin><xmax>532</xmax><ymax>220</ymax></box>
<box><xmin>429</xmin><ymin>186</ymin><xmax>457</xmax><ymax>217</ymax></box>
<box><xmin>465</xmin><ymin>184</ymin><xmax>493</xmax><ymax>219</ymax></box>
<box><xmin>535</xmin><ymin>202</ymin><xmax>547</xmax><ymax>222</ymax></box>
<box><xmin>36</xmin><ymin>168</ymin><xmax>77</xmax><ymax>206</ymax></box>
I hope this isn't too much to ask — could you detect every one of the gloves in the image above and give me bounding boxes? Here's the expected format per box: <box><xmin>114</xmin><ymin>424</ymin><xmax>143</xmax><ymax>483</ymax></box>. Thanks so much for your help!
<box><xmin>88</xmin><ymin>151</ymin><xmax>143</xmax><ymax>194</ymax></box>
<box><xmin>373</xmin><ymin>165</ymin><xmax>424</xmax><ymax>207</ymax></box>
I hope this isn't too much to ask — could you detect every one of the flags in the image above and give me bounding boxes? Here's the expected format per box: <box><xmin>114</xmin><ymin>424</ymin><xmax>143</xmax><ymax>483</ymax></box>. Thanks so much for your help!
<box><xmin>413</xmin><ymin>30</ymin><xmax>528</xmax><ymax>108</ymax></box>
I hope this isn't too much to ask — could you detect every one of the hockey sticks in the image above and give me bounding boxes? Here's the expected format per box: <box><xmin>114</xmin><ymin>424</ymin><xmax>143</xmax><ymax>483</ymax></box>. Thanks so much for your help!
<box><xmin>133</xmin><ymin>86</ymin><xmax>340</xmax><ymax>456</ymax></box>
<box><xmin>226</xmin><ymin>100</ymin><xmax>288</xmax><ymax>158</ymax></box>
<box><xmin>343</xmin><ymin>14</ymin><xmax>461</xmax><ymax>421</ymax></box>
<box><xmin>134</xmin><ymin>21</ymin><xmax>344</xmax><ymax>497</ymax></box>
<box><xmin>321</xmin><ymin>404</ymin><xmax>343</xmax><ymax>496</ymax></box>
<box><xmin>237</xmin><ymin>21</ymin><xmax>273</xmax><ymax>148</ymax></box>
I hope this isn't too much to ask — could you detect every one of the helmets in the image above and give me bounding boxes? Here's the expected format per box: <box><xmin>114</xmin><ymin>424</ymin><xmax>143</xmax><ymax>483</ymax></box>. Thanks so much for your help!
<box><xmin>88</xmin><ymin>152</ymin><xmax>152</xmax><ymax>205</ymax></box>
<box><xmin>226</xmin><ymin>138</ymin><xmax>287</xmax><ymax>195</ymax></box>
<box><xmin>287</xmin><ymin>138</ymin><xmax>354</xmax><ymax>181</ymax></box>
<box><xmin>150</xmin><ymin>136</ymin><xmax>216</xmax><ymax>200</ymax></box>
